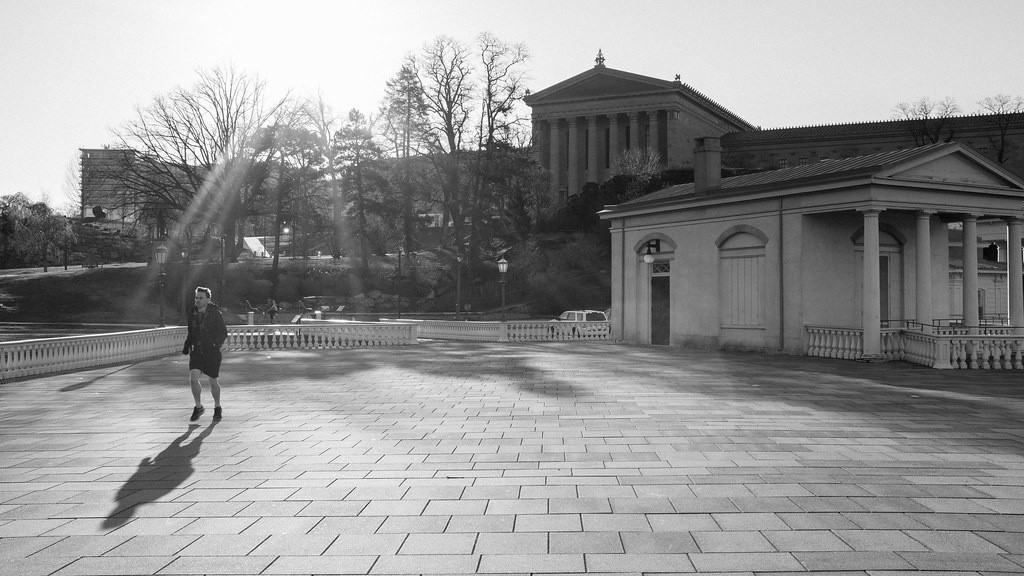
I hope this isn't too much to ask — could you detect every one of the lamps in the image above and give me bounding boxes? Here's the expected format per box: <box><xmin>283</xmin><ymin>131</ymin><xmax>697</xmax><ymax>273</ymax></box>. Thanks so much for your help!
<box><xmin>643</xmin><ymin>238</ymin><xmax>660</xmax><ymax>264</ymax></box>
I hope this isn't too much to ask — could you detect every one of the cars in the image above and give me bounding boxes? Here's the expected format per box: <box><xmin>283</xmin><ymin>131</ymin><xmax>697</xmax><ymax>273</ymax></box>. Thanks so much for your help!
<box><xmin>546</xmin><ymin>309</ymin><xmax>608</xmax><ymax>338</ymax></box>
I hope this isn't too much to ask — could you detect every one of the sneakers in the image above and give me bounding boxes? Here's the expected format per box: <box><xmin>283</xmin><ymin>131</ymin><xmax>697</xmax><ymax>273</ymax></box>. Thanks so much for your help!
<box><xmin>213</xmin><ymin>406</ymin><xmax>222</xmax><ymax>420</ymax></box>
<box><xmin>190</xmin><ymin>404</ymin><xmax>205</xmax><ymax>421</ymax></box>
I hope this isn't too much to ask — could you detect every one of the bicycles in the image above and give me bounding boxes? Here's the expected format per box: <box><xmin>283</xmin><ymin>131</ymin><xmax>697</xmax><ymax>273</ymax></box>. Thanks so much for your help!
<box><xmin>257</xmin><ymin>309</ymin><xmax>284</xmax><ymax>324</ymax></box>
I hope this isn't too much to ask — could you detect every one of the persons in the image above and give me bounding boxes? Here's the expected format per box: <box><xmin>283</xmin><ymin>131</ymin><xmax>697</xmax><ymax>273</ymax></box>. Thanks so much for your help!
<box><xmin>183</xmin><ymin>286</ymin><xmax>227</xmax><ymax>422</ymax></box>
<box><xmin>264</xmin><ymin>298</ymin><xmax>278</xmax><ymax>321</ymax></box>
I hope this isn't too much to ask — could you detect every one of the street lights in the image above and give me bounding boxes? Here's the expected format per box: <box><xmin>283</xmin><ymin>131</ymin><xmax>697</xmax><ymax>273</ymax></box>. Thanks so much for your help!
<box><xmin>396</xmin><ymin>238</ymin><xmax>404</xmax><ymax>320</ymax></box>
<box><xmin>153</xmin><ymin>240</ymin><xmax>168</xmax><ymax>327</ymax></box>
<box><xmin>62</xmin><ymin>216</ymin><xmax>70</xmax><ymax>270</ymax></box>
<box><xmin>220</xmin><ymin>232</ymin><xmax>227</xmax><ymax>304</ymax></box>
<box><xmin>180</xmin><ymin>245</ymin><xmax>189</xmax><ymax>326</ymax></box>
<box><xmin>456</xmin><ymin>252</ymin><xmax>464</xmax><ymax>320</ymax></box>
<box><xmin>496</xmin><ymin>253</ymin><xmax>509</xmax><ymax>322</ymax></box>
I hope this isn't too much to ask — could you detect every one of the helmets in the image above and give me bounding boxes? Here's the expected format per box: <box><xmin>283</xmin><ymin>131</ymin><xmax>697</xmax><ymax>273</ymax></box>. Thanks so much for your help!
<box><xmin>266</xmin><ymin>299</ymin><xmax>272</xmax><ymax>302</ymax></box>
